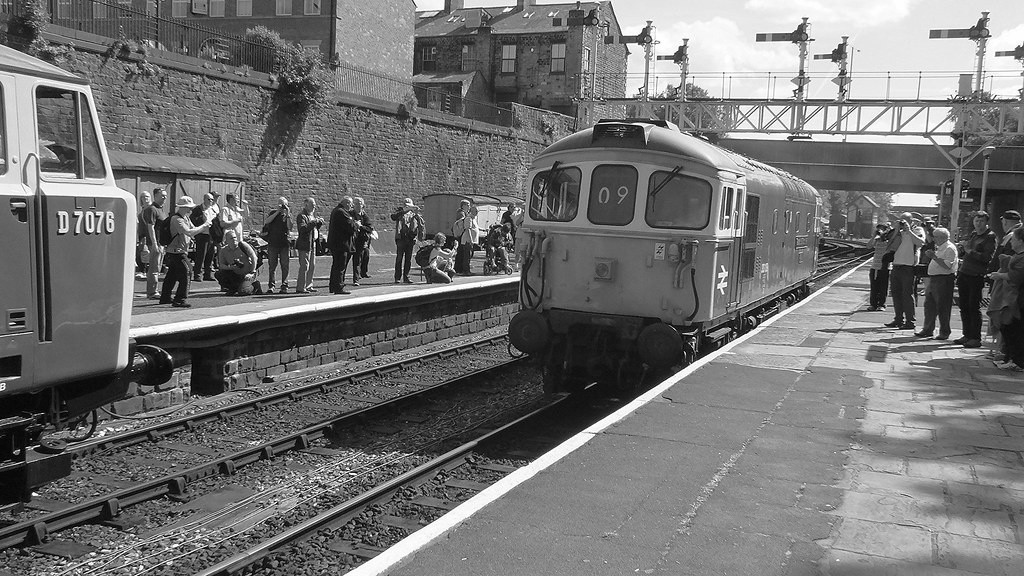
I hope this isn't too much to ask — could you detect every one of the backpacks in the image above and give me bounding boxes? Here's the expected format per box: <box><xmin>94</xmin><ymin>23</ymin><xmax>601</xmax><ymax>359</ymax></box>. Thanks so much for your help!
<box><xmin>159</xmin><ymin>213</ymin><xmax>185</xmax><ymax>245</ymax></box>
<box><xmin>208</xmin><ymin>212</ymin><xmax>224</xmax><ymax>243</ymax></box>
<box><xmin>238</xmin><ymin>240</ymin><xmax>263</xmax><ymax>270</ymax></box>
<box><xmin>453</xmin><ymin>215</ymin><xmax>472</xmax><ymax>238</ymax></box>
<box><xmin>416</xmin><ymin>245</ymin><xmax>441</xmax><ymax>268</ymax></box>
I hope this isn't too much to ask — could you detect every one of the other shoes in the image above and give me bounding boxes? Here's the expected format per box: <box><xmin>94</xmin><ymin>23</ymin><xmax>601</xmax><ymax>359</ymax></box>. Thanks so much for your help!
<box><xmin>269</xmin><ymin>285</ymin><xmax>275</xmax><ymax>293</ymax></box>
<box><xmin>135</xmin><ymin>272</ymin><xmax>147</xmax><ymax>278</ymax></box>
<box><xmin>149</xmin><ymin>293</ymin><xmax>161</xmax><ymax>299</ymax></box>
<box><xmin>296</xmin><ymin>287</ymin><xmax>318</xmax><ymax>293</ymax></box>
<box><xmin>203</xmin><ymin>276</ymin><xmax>215</xmax><ymax>281</ymax></box>
<box><xmin>159</xmin><ymin>296</ymin><xmax>174</xmax><ymax>304</ymax></box>
<box><xmin>353</xmin><ymin>281</ymin><xmax>360</xmax><ymax>285</ymax></box>
<box><xmin>491</xmin><ymin>262</ymin><xmax>497</xmax><ymax>267</ymax></box>
<box><xmin>251</xmin><ymin>281</ymin><xmax>262</xmax><ymax>295</ymax></box>
<box><xmin>504</xmin><ymin>264</ymin><xmax>511</xmax><ymax>269</ymax></box>
<box><xmin>335</xmin><ymin>289</ymin><xmax>352</xmax><ymax>294</ymax></box>
<box><xmin>456</xmin><ymin>271</ymin><xmax>475</xmax><ymax>276</ymax></box>
<box><xmin>867</xmin><ymin>305</ymin><xmax>1024</xmax><ymax>370</ymax></box>
<box><xmin>394</xmin><ymin>279</ymin><xmax>413</xmax><ymax>283</ymax></box>
<box><xmin>279</xmin><ymin>285</ymin><xmax>288</xmax><ymax>294</ymax></box>
<box><xmin>195</xmin><ymin>276</ymin><xmax>202</xmax><ymax>281</ymax></box>
<box><xmin>361</xmin><ymin>273</ymin><xmax>372</xmax><ymax>278</ymax></box>
<box><xmin>173</xmin><ymin>300</ymin><xmax>191</xmax><ymax>307</ymax></box>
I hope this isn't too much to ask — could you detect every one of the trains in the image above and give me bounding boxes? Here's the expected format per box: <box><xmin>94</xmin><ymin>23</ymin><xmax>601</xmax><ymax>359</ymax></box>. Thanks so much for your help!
<box><xmin>0</xmin><ymin>42</ymin><xmax>177</xmax><ymax>457</ymax></box>
<box><xmin>503</xmin><ymin>118</ymin><xmax>821</xmax><ymax>394</ymax></box>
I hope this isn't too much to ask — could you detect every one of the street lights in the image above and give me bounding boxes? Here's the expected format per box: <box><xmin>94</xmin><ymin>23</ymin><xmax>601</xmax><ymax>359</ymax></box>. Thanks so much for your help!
<box><xmin>979</xmin><ymin>145</ymin><xmax>996</xmax><ymax>213</ymax></box>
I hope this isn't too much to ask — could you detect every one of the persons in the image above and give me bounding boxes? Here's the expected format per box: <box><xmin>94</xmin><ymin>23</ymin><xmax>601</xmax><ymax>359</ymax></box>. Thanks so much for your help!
<box><xmin>296</xmin><ymin>197</ymin><xmax>325</xmax><ymax>294</ymax></box>
<box><xmin>985</xmin><ymin>210</ymin><xmax>1024</xmax><ymax>373</ymax></box>
<box><xmin>327</xmin><ymin>196</ymin><xmax>363</xmax><ymax>295</ymax></box>
<box><xmin>953</xmin><ymin>211</ymin><xmax>999</xmax><ymax>349</ymax></box>
<box><xmin>867</xmin><ymin>224</ymin><xmax>890</xmax><ymax>312</ymax></box>
<box><xmin>190</xmin><ymin>190</ymin><xmax>244</xmax><ymax>282</ymax></box>
<box><xmin>489</xmin><ymin>201</ymin><xmax>526</xmax><ymax>272</ymax></box>
<box><xmin>884</xmin><ymin>211</ymin><xmax>925</xmax><ymax>330</ymax></box>
<box><xmin>453</xmin><ymin>200</ymin><xmax>485</xmax><ymax>276</ymax></box>
<box><xmin>138</xmin><ymin>187</ymin><xmax>213</xmax><ymax>308</ymax></box>
<box><xmin>214</xmin><ymin>230</ymin><xmax>263</xmax><ymax>296</ymax></box>
<box><xmin>422</xmin><ymin>232</ymin><xmax>459</xmax><ymax>284</ymax></box>
<box><xmin>265</xmin><ymin>196</ymin><xmax>294</xmax><ymax>294</ymax></box>
<box><xmin>390</xmin><ymin>198</ymin><xmax>425</xmax><ymax>283</ymax></box>
<box><xmin>343</xmin><ymin>197</ymin><xmax>374</xmax><ymax>286</ymax></box>
<box><xmin>914</xmin><ymin>226</ymin><xmax>958</xmax><ymax>340</ymax></box>
<box><xmin>911</xmin><ymin>212</ymin><xmax>937</xmax><ymax>306</ymax></box>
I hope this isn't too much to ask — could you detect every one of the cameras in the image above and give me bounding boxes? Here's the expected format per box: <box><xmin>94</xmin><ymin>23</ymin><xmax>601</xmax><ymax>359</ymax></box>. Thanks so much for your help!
<box><xmin>898</xmin><ymin>220</ymin><xmax>904</xmax><ymax>226</ymax></box>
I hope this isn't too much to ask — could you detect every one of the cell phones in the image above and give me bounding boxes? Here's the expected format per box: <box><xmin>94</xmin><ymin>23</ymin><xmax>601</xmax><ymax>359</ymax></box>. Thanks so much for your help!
<box><xmin>281</xmin><ymin>204</ymin><xmax>287</xmax><ymax>209</ymax></box>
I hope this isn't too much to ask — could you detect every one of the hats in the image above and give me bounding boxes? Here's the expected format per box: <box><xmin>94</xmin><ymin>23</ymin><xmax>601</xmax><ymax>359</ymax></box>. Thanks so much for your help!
<box><xmin>176</xmin><ymin>196</ymin><xmax>196</xmax><ymax>207</ymax></box>
<box><xmin>924</xmin><ymin>217</ymin><xmax>935</xmax><ymax>223</ymax></box>
<box><xmin>1001</xmin><ymin>211</ymin><xmax>1021</xmax><ymax>221</ymax></box>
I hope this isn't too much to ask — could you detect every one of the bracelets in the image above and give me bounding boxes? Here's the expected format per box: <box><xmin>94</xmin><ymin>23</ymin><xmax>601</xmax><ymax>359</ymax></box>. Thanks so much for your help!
<box><xmin>287</xmin><ymin>216</ymin><xmax>290</xmax><ymax>218</ymax></box>
<box><xmin>934</xmin><ymin>257</ymin><xmax>937</xmax><ymax>260</ymax></box>
<box><xmin>250</xmin><ymin>271</ymin><xmax>256</xmax><ymax>273</ymax></box>
